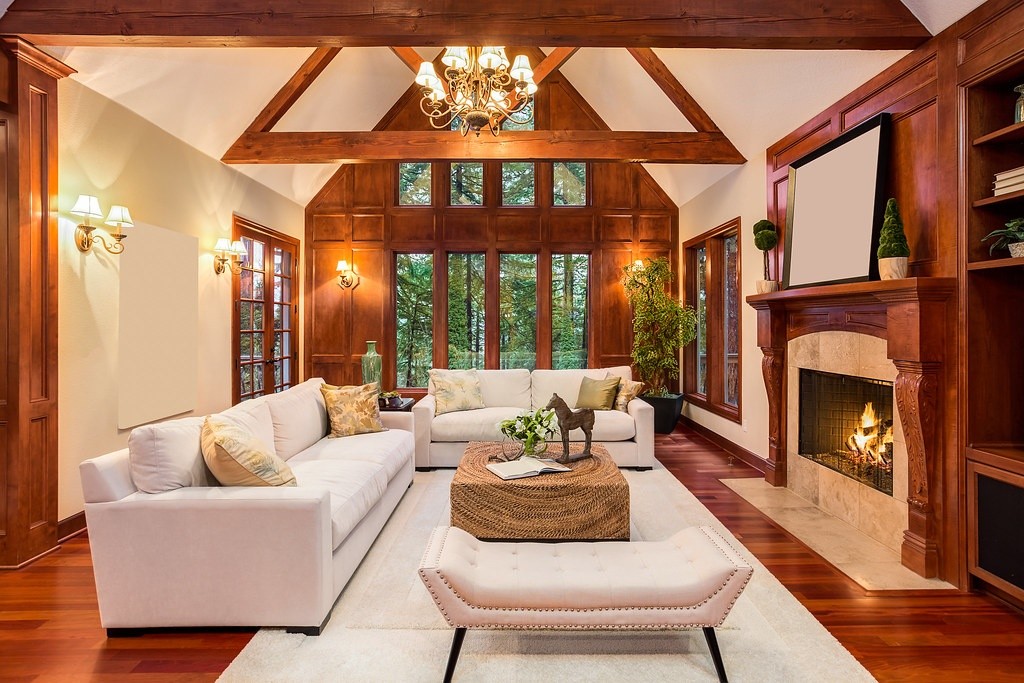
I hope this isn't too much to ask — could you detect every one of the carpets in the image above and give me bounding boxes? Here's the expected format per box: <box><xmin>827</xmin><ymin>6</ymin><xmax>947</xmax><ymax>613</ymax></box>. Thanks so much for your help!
<box><xmin>213</xmin><ymin>456</ymin><xmax>879</xmax><ymax>683</ymax></box>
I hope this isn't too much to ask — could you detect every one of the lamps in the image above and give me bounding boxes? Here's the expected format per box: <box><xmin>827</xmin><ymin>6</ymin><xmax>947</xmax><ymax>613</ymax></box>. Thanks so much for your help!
<box><xmin>414</xmin><ymin>46</ymin><xmax>538</xmax><ymax>136</ymax></box>
<box><xmin>70</xmin><ymin>194</ymin><xmax>133</xmax><ymax>255</ymax></box>
<box><xmin>336</xmin><ymin>260</ymin><xmax>359</xmax><ymax>289</ymax></box>
<box><xmin>213</xmin><ymin>238</ymin><xmax>248</xmax><ymax>274</ymax></box>
<box><xmin>632</xmin><ymin>259</ymin><xmax>646</xmax><ymax>271</ymax></box>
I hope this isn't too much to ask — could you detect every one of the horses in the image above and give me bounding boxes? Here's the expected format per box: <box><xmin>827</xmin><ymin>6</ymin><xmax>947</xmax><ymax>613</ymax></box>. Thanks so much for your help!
<box><xmin>546</xmin><ymin>393</ymin><xmax>595</xmax><ymax>459</ymax></box>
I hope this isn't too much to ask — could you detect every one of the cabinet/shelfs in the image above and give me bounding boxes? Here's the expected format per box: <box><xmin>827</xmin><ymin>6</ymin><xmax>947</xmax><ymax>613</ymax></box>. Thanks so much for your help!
<box><xmin>964</xmin><ymin>119</ymin><xmax>1023</xmax><ymax>611</ymax></box>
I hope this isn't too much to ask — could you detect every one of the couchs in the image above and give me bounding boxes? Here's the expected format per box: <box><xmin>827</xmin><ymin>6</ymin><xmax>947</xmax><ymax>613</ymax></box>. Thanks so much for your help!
<box><xmin>410</xmin><ymin>366</ymin><xmax>655</xmax><ymax>473</ymax></box>
<box><xmin>77</xmin><ymin>376</ymin><xmax>417</xmax><ymax>637</ymax></box>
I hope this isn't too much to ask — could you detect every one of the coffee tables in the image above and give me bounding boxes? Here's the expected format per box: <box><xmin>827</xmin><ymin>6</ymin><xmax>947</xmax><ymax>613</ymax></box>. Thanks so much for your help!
<box><xmin>451</xmin><ymin>441</ymin><xmax>629</xmax><ymax>542</ymax></box>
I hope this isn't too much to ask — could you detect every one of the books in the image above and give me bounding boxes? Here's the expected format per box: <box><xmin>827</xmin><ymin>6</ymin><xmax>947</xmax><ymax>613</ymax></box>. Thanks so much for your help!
<box><xmin>485</xmin><ymin>456</ymin><xmax>573</xmax><ymax>480</ymax></box>
<box><xmin>991</xmin><ymin>165</ymin><xmax>1024</xmax><ymax>196</ymax></box>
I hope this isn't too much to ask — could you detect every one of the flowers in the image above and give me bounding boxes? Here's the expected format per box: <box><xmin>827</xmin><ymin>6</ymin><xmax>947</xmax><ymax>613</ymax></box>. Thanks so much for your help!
<box><xmin>500</xmin><ymin>406</ymin><xmax>562</xmax><ymax>456</ymax></box>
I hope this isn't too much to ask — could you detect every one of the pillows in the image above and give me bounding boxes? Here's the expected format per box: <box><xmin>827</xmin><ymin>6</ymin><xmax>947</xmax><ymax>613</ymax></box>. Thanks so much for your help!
<box><xmin>575</xmin><ymin>377</ymin><xmax>620</xmax><ymax>409</ymax></box>
<box><xmin>319</xmin><ymin>382</ymin><xmax>388</xmax><ymax>439</ymax></box>
<box><xmin>199</xmin><ymin>414</ymin><xmax>297</xmax><ymax>485</ymax></box>
<box><xmin>432</xmin><ymin>368</ymin><xmax>486</xmax><ymax>415</ymax></box>
<box><xmin>604</xmin><ymin>372</ymin><xmax>645</xmax><ymax>414</ymax></box>
<box><xmin>322</xmin><ymin>383</ymin><xmax>358</xmax><ymax>433</ymax></box>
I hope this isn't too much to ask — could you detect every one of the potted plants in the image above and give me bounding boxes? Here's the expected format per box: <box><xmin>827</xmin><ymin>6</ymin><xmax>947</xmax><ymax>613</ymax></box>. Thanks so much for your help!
<box><xmin>382</xmin><ymin>390</ymin><xmax>401</xmax><ymax>407</ymax></box>
<box><xmin>753</xmin><ymin>220</ymin><xmax>778</xmax><ymax>294</ymax></box>
<box><xmin>981</xmin><ymin>218</ymin><xmax>1024</xmax><ymax>259</ymax></box>
<box><xmin>620</xmin><ymin>256</ymin><xmax>696</xmax><ymax>434</ymax></box>
<box><xmin>876</xmin><ymin>199</ymin><xmax>910</xmax><ymax>279</ymax></box>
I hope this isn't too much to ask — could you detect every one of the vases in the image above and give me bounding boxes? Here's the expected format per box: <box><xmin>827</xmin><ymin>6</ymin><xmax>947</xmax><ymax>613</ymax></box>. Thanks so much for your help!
<box><xmin>363</xmin><ymin>341</ymin><xmax>382</xmax><ymax>396</ymax></box>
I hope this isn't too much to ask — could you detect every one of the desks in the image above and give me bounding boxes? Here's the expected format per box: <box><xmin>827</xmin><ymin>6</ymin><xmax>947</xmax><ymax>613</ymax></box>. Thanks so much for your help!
<box><xmin>379</xmin><ymin>397</ymin><xmax>415</xmax><ymax>412</ymax></box>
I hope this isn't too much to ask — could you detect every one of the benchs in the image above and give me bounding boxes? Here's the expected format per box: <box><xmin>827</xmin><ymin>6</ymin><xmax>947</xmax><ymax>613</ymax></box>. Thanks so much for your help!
<box><xmin>420</xmin><ymin>526</ymin><xmax>755</xmax><ymax>683</ymax></box>
